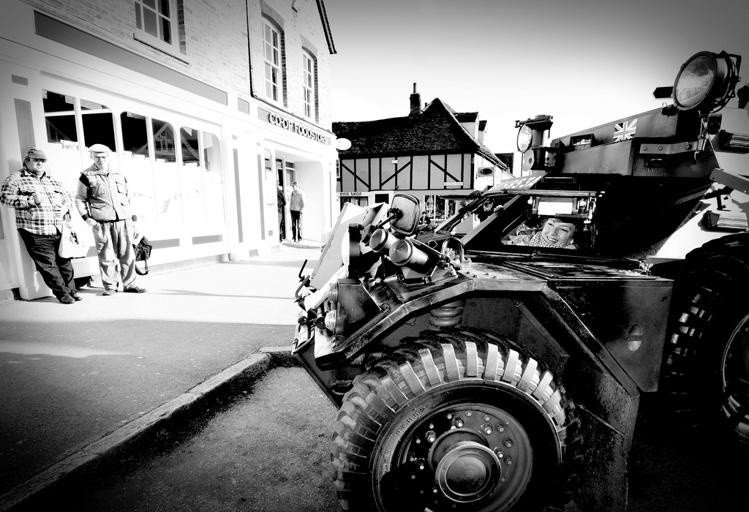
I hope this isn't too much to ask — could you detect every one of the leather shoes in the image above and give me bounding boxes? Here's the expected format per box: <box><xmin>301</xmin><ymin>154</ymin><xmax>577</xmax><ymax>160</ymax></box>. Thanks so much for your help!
<box><xmin>53</xmin><ymin>289</ymin><xmax>83</xmax><ymax>304</ymax></box>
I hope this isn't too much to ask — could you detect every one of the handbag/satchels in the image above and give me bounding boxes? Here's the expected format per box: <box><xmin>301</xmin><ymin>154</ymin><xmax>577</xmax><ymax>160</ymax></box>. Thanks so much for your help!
<box><xmin>58</xmin><ymin>220</ymin><xmax>91</xmax><ymax>259</ymax></box>
<box><xmin>134</xmin><ymin>236</ymin><xmax>152</xmax><ymax>261</ymax></box>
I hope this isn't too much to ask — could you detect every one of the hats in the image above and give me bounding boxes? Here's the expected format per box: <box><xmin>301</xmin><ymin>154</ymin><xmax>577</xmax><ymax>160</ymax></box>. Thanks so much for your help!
<box><xmin>25</xmin><ymin>147</ymin><xmax>46</xmax><ymax>160</ymax></box>
<box><xmin>87</xmin><ymin>143</ymin><xmax>112</xmax><ymax>153</ymax></box>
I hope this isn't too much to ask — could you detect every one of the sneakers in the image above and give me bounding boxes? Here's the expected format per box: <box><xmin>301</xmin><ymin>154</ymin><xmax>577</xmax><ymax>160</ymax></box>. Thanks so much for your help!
<box><xmin>102</xmin><ymin>285</ymin><xmax>146</xmax><ymax>296</ymax></box>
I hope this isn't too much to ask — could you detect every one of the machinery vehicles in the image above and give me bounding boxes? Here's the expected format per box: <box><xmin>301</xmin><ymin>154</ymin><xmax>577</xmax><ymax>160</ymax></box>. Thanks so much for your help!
<box><xmin>291</xmin><ymin>48</ymin><xmax>749</xmax><ymax>511</ymax></box>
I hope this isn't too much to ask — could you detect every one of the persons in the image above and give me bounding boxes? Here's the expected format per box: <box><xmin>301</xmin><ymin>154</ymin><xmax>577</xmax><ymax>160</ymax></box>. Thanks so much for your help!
<box><xmin>277</xmin><ymin>181</ymin><xmax>304</xmax><ymax>242</ymax></box>
<box><xmin>0</xmin><ymin>143</ymin><xmax>151</xmax><ymax>304</ymax></box>
<box><xmin>506</xmin><ymin>211</ymin><xmax>585</xmax><ymax>249</ymax></box>
